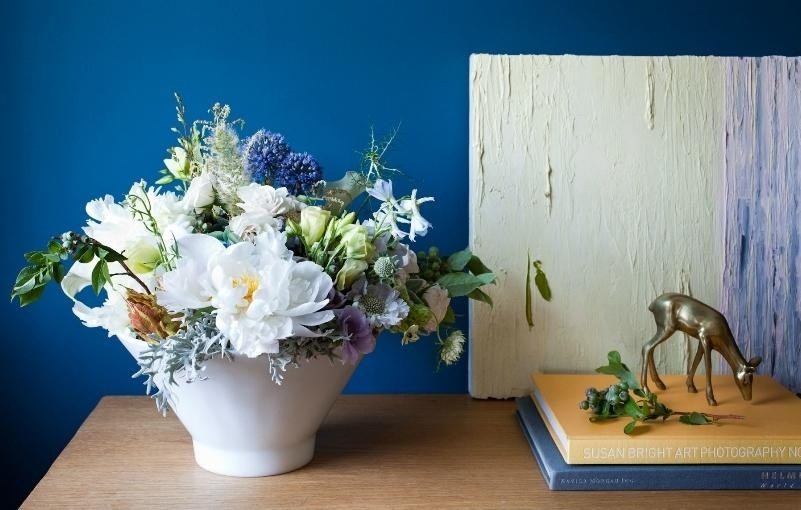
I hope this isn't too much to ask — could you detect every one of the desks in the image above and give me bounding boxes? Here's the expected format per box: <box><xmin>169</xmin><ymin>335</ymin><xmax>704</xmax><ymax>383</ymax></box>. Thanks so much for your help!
<box><xmin>18</xmin><ymin>392</ymin><xmax>800</xmax><ymax>510</ymax></box>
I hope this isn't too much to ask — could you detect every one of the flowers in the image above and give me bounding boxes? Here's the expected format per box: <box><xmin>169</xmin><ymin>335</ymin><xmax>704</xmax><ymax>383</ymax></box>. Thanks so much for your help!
<box><xmin>12</xmin><ymin>101</ymin><xmax>501</xmax><ymax>413</ymax></box>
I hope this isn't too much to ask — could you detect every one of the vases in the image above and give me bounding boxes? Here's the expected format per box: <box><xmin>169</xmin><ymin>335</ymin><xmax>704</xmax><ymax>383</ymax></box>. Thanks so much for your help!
<box><xmin>100</xmin><ymin>332</ymin><xmax>376</xmax><ymax>481</ymax></box>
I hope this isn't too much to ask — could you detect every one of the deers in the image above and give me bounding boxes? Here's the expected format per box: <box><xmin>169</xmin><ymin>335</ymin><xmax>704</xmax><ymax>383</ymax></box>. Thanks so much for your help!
<box><xmin>639</xmin><ymin>288</ymin><xmax>764</xmax><ymax>408</ymax></box>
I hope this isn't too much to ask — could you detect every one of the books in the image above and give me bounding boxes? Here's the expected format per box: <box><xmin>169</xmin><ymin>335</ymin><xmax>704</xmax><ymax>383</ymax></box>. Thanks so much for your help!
<box><xmin>528</xmin><ymin>373</ymin><xmax>801</xmax><ymax>466</ymax></box>
<box><xmin>516</xmin><ymin>394</ymin><xmax>801</xmax><ymax>491</ymax></box>
